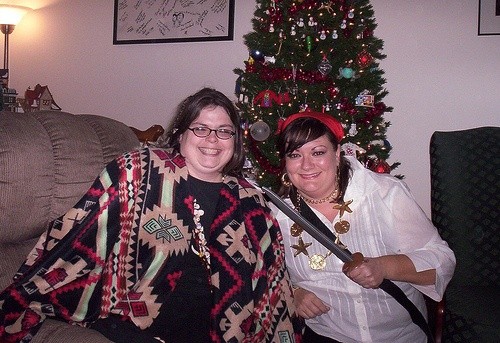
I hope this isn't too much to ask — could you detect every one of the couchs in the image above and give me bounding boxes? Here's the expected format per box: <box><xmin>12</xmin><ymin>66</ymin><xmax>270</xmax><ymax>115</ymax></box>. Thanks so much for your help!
<box><xmin>0</xmin><ymin>111</ymin><xmax>165</xmax><ymax>343</ymax></box>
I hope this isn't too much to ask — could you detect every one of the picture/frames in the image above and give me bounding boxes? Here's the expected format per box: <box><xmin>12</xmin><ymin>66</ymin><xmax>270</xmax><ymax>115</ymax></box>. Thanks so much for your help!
<box><xmin>478</xmin><ymin>0</ymin><xmax>500</xmax><ymax>36</ymax></box>
<box><xmin>113</xmin><ymin>0</ymin><xmax>234</xmax><ymax>44</ymax></box>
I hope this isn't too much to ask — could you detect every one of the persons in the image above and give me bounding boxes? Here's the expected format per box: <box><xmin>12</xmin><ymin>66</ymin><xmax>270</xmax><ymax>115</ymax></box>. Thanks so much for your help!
<box><xmin>267</xmin><ymin>111</ymin><xmax>458</xmax><ymax>343</ymax></box>
<box><xmin>49</xmin><ymin>88</ymin><xmax>303</xmax><ymax>343</ymax></box>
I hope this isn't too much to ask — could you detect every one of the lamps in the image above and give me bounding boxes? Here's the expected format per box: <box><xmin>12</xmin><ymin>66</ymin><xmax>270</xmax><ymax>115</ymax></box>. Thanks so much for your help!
<box><xmin>0</xmin><ymin>24</ymin><xmax>16</xmax><ymax>88</ymax></box>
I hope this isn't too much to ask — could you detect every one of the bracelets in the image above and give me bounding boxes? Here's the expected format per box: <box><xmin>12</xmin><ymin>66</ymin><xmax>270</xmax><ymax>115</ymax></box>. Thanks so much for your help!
<box><xmin>293</xmin><ymin>284</ymin><xmax>300</xmax><ymax>296</ymax></box>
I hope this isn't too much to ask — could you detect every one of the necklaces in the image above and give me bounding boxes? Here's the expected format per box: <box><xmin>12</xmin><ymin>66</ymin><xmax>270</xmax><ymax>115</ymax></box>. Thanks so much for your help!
<box><xmin>221</xmin><ymin>174</ymin><xmax>227</xmax><ymax>182</ymax></box>
<box><xmin>290</xmin><ymin>179</ymin><xmax>354</xmax><ymax>271</ymax></box>
<box><xmin>298</xmin><ymin>185</ymin><xmax>339</xmax><ymax>204</ymax></box>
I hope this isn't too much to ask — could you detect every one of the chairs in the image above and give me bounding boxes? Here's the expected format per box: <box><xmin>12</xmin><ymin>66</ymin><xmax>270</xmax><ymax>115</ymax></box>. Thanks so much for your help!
<box><xmin>423</xmin><ymin>127</ymin><xmax>500</xmax><ymax>343</ymax></box>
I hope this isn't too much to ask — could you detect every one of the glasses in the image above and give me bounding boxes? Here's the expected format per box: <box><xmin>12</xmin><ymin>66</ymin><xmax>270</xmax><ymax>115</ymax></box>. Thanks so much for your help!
<box><xmin>187</xmin><ymin>127</ymin><xmax>237</xmax><ymax>139</ymax></box>
